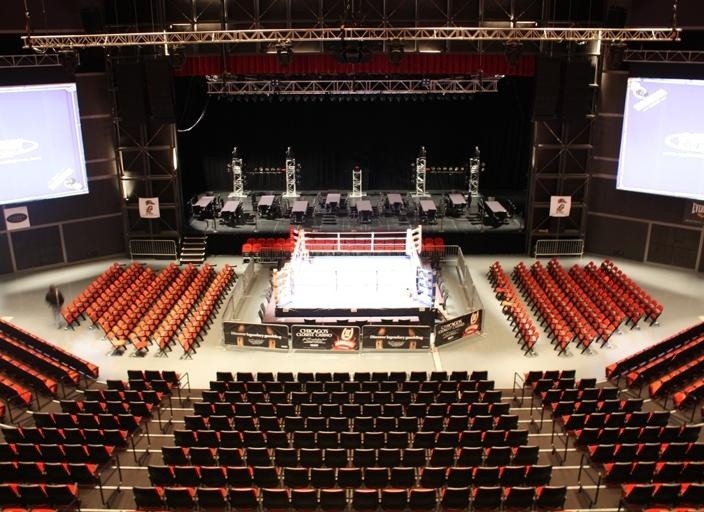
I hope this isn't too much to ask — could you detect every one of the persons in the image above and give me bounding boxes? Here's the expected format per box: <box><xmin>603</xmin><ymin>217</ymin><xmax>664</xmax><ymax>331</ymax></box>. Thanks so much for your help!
<box><xmin>45</xmin><ymin>282</ymin><xmax>65</xmax><ymax>331</ymax></box>
